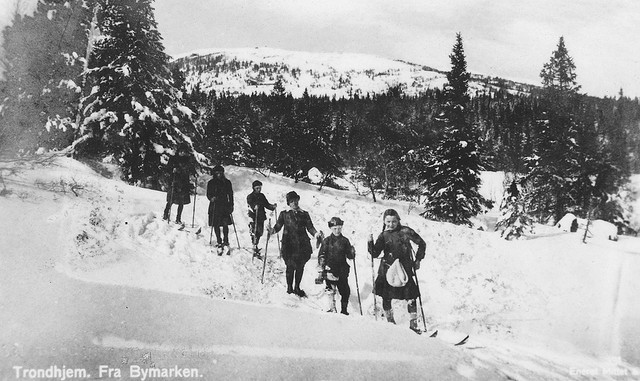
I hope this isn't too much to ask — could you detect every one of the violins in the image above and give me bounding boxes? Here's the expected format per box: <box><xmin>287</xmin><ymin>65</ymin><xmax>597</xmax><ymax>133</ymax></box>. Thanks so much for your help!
<box><xmin>428</xmin><ymin>329</ymin><xmax>469</xmax><ymax>344</ymax></box>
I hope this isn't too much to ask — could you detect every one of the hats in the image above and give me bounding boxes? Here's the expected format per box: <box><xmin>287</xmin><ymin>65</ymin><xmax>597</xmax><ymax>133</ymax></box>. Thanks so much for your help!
<box><xmin>327</xmin><ymin>216</ymin><xmax>344</xmax><ymax>227</ymax></box>
<box><xmin>385</xmin><ymin>259</ymin><xmax>409</xmax><ymax>288</ymax></box>
<box><xmin>175</xmin><ymin>142</ymin><xmax>190</xmax><ymax>152</ymax></box>
<box><xmin>286</xmin><ymin>190</ymin><xmax>300</xmax><ymax>204</ymax></box>
<box><xmin>213</xmin><ymin>164</ymin><xmax>224</xmax><ymax>173</ymax></box>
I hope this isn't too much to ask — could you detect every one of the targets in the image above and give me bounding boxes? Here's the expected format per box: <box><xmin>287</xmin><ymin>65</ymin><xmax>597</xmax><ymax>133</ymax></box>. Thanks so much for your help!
<box><xmin>217</xmin><ymin>246</ymin><xmax>231</xmax><ymax>256</ymax></box>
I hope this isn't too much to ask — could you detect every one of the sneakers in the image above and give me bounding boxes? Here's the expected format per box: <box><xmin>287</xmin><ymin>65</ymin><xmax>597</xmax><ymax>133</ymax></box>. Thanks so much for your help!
<box><xmin>287</xmin><ymin>286</ymin><xmax>294</xmax><ymax>295</ymax></box>
<box><xmin>217</xmin><ymin>240</ymin><xmax>223</xmax><ymax>248</ymax></box>
<box><xmin>409</xmin><ymin>326</ymin><xmax>421</xmax><ymax>334</ymax></box>
<box><xmin>224</xmin><ymin>239</ymin><xmax>229</xmax><ymax>247</ymax></box>
<box><xmin>294</xmin><ymin>286</ymin><xmax>306</xmax><ymax>298</ymax></box>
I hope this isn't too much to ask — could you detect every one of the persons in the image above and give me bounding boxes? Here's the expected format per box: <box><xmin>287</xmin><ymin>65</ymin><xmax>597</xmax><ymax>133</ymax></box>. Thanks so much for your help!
<box><xmin>368</xmin><ymin>209</ymin><xmax>426</xmax><ymax>334</ymax></box>
<box><xmin>266</xmin><ymin>191</ymin><xmax>323</xmax><ymax>297</ymax></box>
<box><xmin>318</xmin><ymin>217</ymin><xmax>355</xmax><ymax>315</ymax></box>
<box><xmin>207</xmin><ymin>166</ymin><xmax>234</xmax><ymax>248</ymax></box>
<box><xmin>247</xmin><ymin>180</ymin><xmax>277</xmax><ymax>250</ymax></box>
<box><xmin>163</xmin><ymin>143</ymin><xmax>195</xmax><ymax>225</ymax></box>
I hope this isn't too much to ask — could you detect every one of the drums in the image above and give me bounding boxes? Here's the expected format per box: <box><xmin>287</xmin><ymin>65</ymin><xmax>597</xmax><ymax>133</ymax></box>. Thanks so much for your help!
<box><xmin>177</xmin><ymin>223</ymin><xmax>201</xmax><ymax>234</ymax></box>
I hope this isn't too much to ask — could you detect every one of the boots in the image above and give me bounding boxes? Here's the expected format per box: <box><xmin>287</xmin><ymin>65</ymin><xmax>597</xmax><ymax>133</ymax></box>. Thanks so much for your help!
<box><xmin>341</xmin><ymin>301</ymin><xmax>349</xmax><ymax>316</ymax></box>
